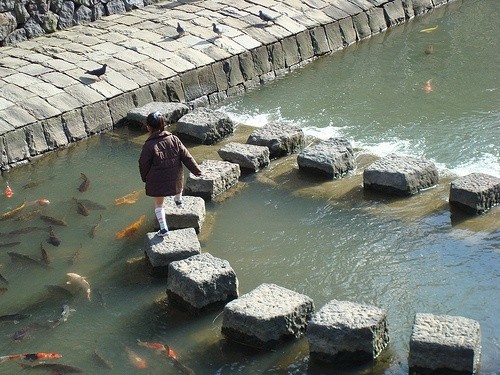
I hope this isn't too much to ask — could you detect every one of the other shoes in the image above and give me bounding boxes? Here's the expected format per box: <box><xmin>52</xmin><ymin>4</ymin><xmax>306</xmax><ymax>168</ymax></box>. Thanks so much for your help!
<box><xmin>157</xmin><ymin>228</ymin><xmax>169</xmax><ymax>237</ymax></box>
<box><xmin>174</xmin><ymin>197</ymin><xmax>184</xmax><ymax>207</ymax></box>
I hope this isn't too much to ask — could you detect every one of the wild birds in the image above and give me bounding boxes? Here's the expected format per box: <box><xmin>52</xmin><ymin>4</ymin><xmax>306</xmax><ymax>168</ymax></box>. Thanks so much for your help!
<box><xmin>212</xmin><ymin>23</ymin><xmax>224</xmax><ymax>35</ymax></box>
<box><xmin>176</xmin><ymin>22</ymin><xmax>186</xmax><ymax>35</ymax></box>
<box><xmin>84</xmin><ymin>64</ymin><xmax>107</xmax><ymax>79</ymax></box>
<box><xmin>259</xmin><ymin>10</ymin><xmax>276</xmax><ymax>25</ymax></box>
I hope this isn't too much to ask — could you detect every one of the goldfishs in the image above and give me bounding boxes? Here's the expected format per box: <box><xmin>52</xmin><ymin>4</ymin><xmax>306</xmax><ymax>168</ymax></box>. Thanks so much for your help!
<box><xmin>419</xmin><ymin>25</ymin><xmax>438</xmax><ymax>34</ymax></box>
<box><xmin>422</xmin><ymin>79</ymin><xmax>433</xmax><ymax>94</ymax></box>
<box><xmin>0</xmin><ymin>167</ymin><xmax>195</xmax><ymax>375</ymax></box>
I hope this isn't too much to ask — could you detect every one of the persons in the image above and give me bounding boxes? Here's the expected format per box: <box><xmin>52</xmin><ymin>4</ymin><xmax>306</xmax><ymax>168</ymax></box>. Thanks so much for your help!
<box><xmin>139</xmin><ymin>112</ymin><xmax>201</xmax><ymax>237</ymax></box>
